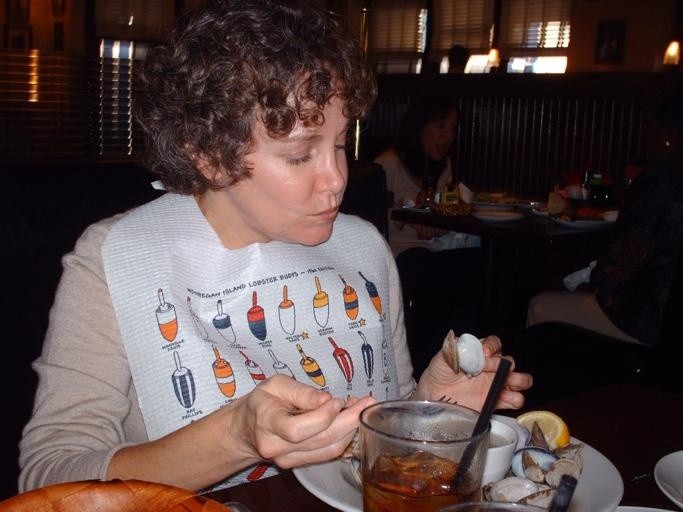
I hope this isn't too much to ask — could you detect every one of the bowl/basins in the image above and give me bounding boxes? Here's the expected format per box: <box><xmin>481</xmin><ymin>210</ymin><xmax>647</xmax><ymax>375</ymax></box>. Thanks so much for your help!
<box><xmin>481</xmin><ymin>419</ymin><xmax>521</xmax><ymax>486</ymax></box>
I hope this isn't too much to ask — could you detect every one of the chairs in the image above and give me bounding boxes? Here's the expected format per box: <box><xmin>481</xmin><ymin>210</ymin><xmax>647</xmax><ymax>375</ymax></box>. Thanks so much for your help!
<box><xmin>528</xmin><ymin>253</ymin><xmax>683</xmax><ymax>396</ymax></box>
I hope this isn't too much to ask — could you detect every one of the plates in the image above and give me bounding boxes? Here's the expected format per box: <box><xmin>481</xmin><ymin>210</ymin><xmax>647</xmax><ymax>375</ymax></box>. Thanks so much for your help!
<box><xmin>289</xmin><ymin>412</ymin><xmax>627</xmax><ymax>512</ymax></box>
<box><xmin>468</xmin><ymin>198</ymin><xmax>520</xmax><ymax>213</ymax></box>
<box><xmin>530</xmin><ymin>208</ymin><xmax>553</xmax><ymax>218</ymax></box>
<box><xmin>470</xmin><ymin>210</ymin><xmax>526</xmax><ymax>224</ymax></box>
<box><xmin>653</xmin><ymin>446</ymin><xmax>683</xmax><ymax>509</ymax></box>
<box><xmin>553</xmin><ymin>215</ymin><xmax>609</xmax><ymax>230</ymax></box>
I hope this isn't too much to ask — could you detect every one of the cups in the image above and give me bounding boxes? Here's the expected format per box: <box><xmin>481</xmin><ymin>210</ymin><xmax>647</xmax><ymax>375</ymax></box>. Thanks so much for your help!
<box><xmin>357</xmin><ymin>397</ymin><xmax>491</xmax><ymax>511</ymax></box>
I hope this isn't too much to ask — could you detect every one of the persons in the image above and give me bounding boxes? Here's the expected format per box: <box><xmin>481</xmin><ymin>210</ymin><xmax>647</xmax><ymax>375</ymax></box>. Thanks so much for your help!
<box><xmin>11</xmin><ymin>2</ymin><xmax>533</xmax><ymax>493</ymax></box>
<box><xmin>373</xmin><ymin>76</ymin><xmax>498</xmax><ymax>332</ymax></box>
<box><xmin>526</xmin><ymin>86</ymin><xmax>681</xmax><ymax>348</ymax></box>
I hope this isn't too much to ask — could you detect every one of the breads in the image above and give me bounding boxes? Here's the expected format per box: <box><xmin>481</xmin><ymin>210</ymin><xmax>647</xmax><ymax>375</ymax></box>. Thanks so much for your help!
<box><xmin>0</xmin><ymin>478</ymin><xmax>232</xmax><ymax>512</ymax></box>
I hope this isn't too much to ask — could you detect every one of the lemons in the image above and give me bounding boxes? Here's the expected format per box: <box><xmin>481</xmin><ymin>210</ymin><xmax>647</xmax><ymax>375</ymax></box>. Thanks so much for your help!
<box><xmin>517</xmin><ymin>410</ymin><xmax>570</xmax><ymax>453</ymax></box>
